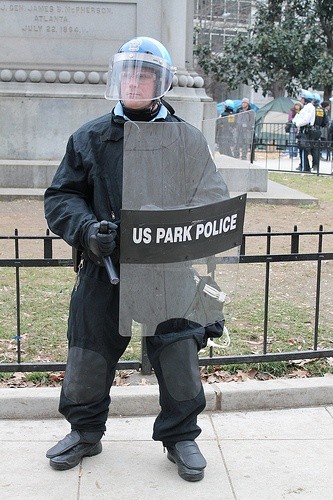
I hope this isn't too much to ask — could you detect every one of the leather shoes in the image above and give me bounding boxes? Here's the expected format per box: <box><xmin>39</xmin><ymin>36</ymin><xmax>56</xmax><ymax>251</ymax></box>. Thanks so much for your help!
<box><xmin>167</xmin><ymin>440</ymin><xmax>207</xmax><ymax>481</ymax></box>
<box><xmin>45</xmin><ymin>431</ymin><xmax>102</xmax><ymax>470</ymax></box>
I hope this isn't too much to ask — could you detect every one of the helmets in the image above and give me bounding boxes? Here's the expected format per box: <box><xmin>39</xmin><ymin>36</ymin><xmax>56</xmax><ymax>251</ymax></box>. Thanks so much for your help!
<box><xmin>312</xmin><ymin>93</ymin><xmax>321</xmax><ymax>100</ymax></box>
<box><xmin>224</xmin><ymin>99</ymin><xmax>234</xmax><ymax>109</ymax></box>
<box><xmin>301</xmin><ymin>89</ymin><xmax>314</xmax><ymax>99</ymax></box>
<box><xmin>104</xmin><ymin>37</ymin><xmax>177</xmax><ymax>101</ymax></box>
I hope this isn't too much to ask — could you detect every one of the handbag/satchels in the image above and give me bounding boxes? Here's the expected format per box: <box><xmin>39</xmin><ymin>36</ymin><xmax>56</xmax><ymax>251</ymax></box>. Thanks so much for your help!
<box><xmin>198</xmin><ymin>275</ymin><xmax>224</xmax><ymax>338</ymax></box>
<box><xmin>285</xmin><ymin>121</ymin><xmax>292</xmax><ymax>132</ymax></box>
<box><xmin>298</xmin><ymin>134</ymin><xmax>311</xmax><ymax>148</ymax></box>
<box><xmin>308</xmin><ymin>128</ymin><xmax>322</xmax><ymax>141</ymax></box>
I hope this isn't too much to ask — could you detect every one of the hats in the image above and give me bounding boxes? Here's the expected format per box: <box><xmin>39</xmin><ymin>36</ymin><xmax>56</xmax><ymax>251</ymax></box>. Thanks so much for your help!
<box><xmin>241</xmin><ymin>97</ymin><xmax>249</xmax><ymax>104</ymax></box>
<box><xmin>321</xmin><ymin>101</ymin><xmax>330</xmax><ymax>108</ymax></box>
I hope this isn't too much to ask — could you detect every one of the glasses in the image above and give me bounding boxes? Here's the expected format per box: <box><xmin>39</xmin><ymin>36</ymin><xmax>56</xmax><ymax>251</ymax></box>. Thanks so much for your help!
<box><xmin>118</xmin><ymin>71</ymin><xmax>156</xmax><ymax>85</ymax></box>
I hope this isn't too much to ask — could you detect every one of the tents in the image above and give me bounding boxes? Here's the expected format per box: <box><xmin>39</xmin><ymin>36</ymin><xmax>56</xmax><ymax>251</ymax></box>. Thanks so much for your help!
<box><xmin>215</xmin><ymin>96</ymin><xmax>296</xmax><ymax>150</ymax></box>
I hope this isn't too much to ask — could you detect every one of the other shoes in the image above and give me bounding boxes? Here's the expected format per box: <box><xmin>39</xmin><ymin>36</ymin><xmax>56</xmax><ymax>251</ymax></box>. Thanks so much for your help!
<box><xmin>300</xmin><ymin>168</ymin><xmax>309</xmax><ymax>171</ymax></box>
<box><xmin>313</xmin><ymin>165</ymin><xmax>317</xmax><ymax>171</ymax></box>
<box><xmin>296</xmin><ymin>166</ymin><xmax>301</xmax><ymax>170</ymax></box>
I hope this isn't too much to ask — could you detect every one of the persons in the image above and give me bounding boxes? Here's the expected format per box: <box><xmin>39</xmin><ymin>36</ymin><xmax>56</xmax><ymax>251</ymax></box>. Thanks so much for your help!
<box><xmin>45</xmin><ymin>38</ymin><xmax>229</xmax><ymax>483</ymax></box>
<box><xmin>221</xmin><ymin>98</ymin><xmax>255</xmax><ymax>160</ymax></box>
<box><xmin>289</xmin><ymin>90</ymin><xmax>333</xmax><ymax>172</ymax></box>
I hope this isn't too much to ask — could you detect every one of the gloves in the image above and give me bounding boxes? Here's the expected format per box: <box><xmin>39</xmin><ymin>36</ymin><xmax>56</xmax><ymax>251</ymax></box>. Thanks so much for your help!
<box><xmin>84</xmin><ymin>221</ymin><xmax>118</xmax><ymax>257</ymax></box>
